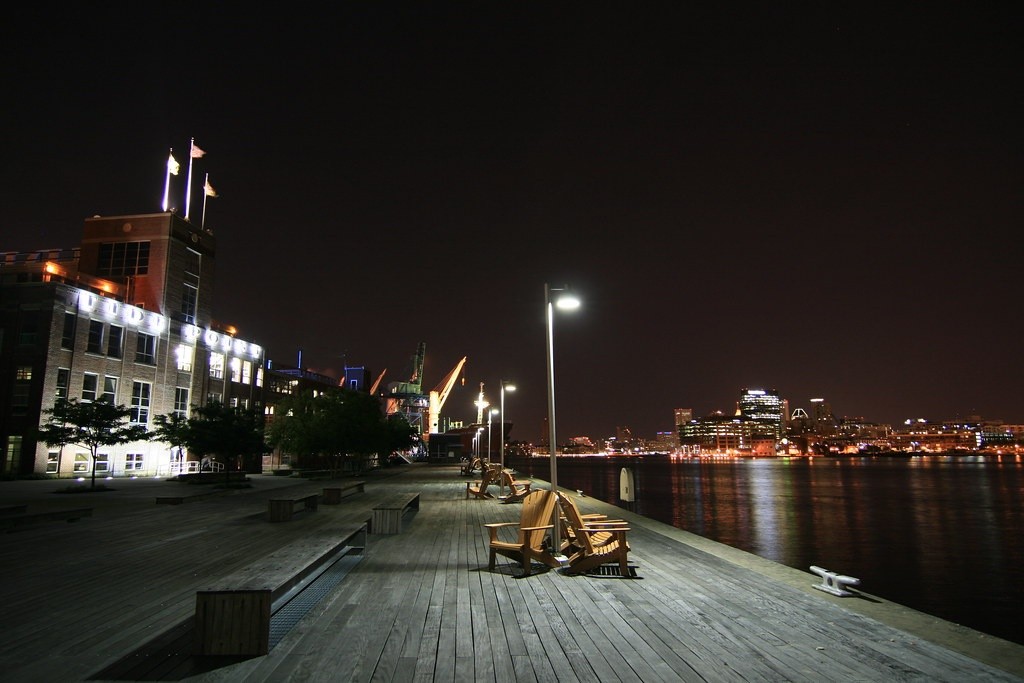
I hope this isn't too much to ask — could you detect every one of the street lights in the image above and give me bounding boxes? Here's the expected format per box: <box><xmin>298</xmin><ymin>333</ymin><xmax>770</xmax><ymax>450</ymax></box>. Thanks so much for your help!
<box><xmin>487</xmin><ymin>406</ymin><xmax>500</xmax><ymax>462</ymax></box>
<box><xmin>543</xmin><ymin>281</ymin><xmax>584</xmax><ymax>562</ymax></box>
<box><xmin>498</xmin><ymin>380</ymin><xmax>518</xmax><ymax>500</ymax></box>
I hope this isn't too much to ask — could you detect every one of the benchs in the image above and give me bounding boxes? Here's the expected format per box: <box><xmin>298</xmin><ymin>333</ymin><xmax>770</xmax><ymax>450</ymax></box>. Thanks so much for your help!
<box><xmin>269</xmin><ymin>492</ymin><xmax>321</xmax><ymax>520</ymax></box>
<box><xmin>193</xmin><ymin>522</ymin><xmax>366</xmax><ymax>656</ymax></box>
<box><xmin>371</xmin><ymin>492</ymin><xmax>420</xmax><ymax>534</ymax></box>
<box><xmin>323</xmin><ymin>481</ymin><xmax>365</xmax><ymax>501</ymax></box>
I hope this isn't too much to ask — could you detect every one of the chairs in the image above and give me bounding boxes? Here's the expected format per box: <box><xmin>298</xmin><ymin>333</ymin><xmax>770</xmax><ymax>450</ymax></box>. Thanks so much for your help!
<box><xmin>460</xmin><ymin>457</ymin><xmax>502</xmax><ymax>479</ymax></box>
<box><xmin>467</xmin><ymin>468</ymin><xmax>494</xmax><ymax>499</ymax></box>
<box><xmin>557</xmin><ymin>491</ymin><xmax>631</xmax><ymax>576</ymax></box>
<box><xmin>502</xmin><ymin>471</ymin><xmax>531</xmax><ymax>502</ymax></box>
<box><xmin>484</xmin><ymin>490</ymin><xmax>560</xmax><ymax>575</ymax></box>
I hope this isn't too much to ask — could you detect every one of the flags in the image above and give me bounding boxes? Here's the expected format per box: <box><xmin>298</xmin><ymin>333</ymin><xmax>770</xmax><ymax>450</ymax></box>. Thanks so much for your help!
<box><xmin>206</xmin><ymin>183</ymin><xmax>218</xmax><ymax>196</ymax></box>
<box><xmin>169</xmin><ymin>155</ymin><xmax>179</xmax><ymax>175</ymax></box>
<box><xmin>193</xmin><ymin>146</ymin><xmax>205</xmax><ymax>158</ymax></box>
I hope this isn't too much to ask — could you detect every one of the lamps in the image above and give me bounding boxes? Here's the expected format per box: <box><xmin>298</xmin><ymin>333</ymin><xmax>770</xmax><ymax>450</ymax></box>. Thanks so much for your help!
<box><xmin>557</xmin><ymin>283</ymin><xmax>579</xmax><ymax>310</ymax></box>
